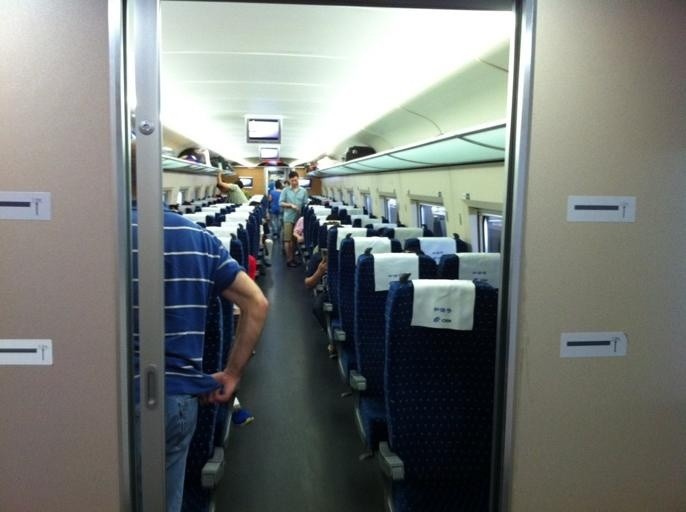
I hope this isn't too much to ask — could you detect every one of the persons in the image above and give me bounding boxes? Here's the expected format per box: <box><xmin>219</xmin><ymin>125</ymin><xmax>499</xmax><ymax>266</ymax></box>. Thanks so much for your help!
<box><xmin>267</xmin><ymin>179</ymin><xmax>283</xmax><ymax>242</ymax></box>
<box><xmin>127</xmin><ymin>137</ymin><xmax>272</xmax><ymax>512</ymax></box>
<box><xmin>326</xmin><ymin>214</ymin><xmax>344</xmax><ymax>221</ymax></box>
<box><xmin>240</xmin><ymin>187</ymin><xmax>252</xmax><ymax>200</ymax></box>
<box><xmin>277</xmin><ymin>170</ymin><xmax>309</xmax><ymax>268</ymax></box>
<box><xmin>215</xmin><ymin>170</ymin><xmax>248</xmax><ymax>203</ymax></box>
<box><xmin>231</xmin><ymin>397</ymin><xmax>255</xmax><ymax>429</ymax></box>
<box><xmin>283</xmin><ymin>180</ymin><xmax>290</xmax><ymax>187</ymax></box>
<box><xmin>312</xmin><ymin>222</ymin><xmax>341</xmax><ymax>254</ymax></box>
<box><xmin>290</xmin><ymin>199</ymin><xmax>319</xmax><ymax>244</ymax></box>
<box><xmin>301</xmin><ymin>225</ymin><xmax>343</xmax><ymax>360</ymax></box>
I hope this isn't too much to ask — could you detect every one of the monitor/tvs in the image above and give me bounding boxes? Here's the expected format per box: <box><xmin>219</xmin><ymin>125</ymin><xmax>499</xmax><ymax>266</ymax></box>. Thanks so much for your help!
<box><xmin>246</xmin><ymin>118</ymin><xmax>281</xmax><ymax>145</ymax></box>
<box><xmin>299</xmin><ymin>177</ymin><xmax>312</xmax><ymax>190</ymax></box>
<box><xmin>239</xmin><ymin>177</ymin><xmax>254</xmax><ymax>189</ymax></box>
<box><xmin>259</xmin><ymin>146</ymin><xmax>280</xmax><ymax>161</ymax></box>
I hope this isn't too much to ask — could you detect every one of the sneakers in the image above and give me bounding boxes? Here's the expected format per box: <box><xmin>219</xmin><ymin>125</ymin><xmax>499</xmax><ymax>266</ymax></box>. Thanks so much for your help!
<box><xmin>230</xmin><ymin>408</ymin><xmax>254</xmax><ymax>428</ymax></box>
<box><xmin>286</xmin><ymin>258</ymin><xmax>302</xmax><ymax>268</ymax></box>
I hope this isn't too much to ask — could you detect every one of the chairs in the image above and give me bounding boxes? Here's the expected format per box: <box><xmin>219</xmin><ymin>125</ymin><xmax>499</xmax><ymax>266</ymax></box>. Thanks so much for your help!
<box><xmin>291</xmin><ymin>184</ymin><xmax>504</xmax><ymax>510</ymax></box>
<box><xmin>169</xmin><ymin>184</ymin><xmax>278</xmax><ymax>509</ymax></box>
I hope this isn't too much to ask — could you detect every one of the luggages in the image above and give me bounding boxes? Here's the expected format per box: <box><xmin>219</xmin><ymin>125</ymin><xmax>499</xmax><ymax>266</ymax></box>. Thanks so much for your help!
<box><xmin>345</xmin><ymin>146</ymin><xmax>375</xmax><ymax>162</ymax></box>
<box><xmin>306</xmin><ymin>166</ymin><xmax>317</xmax><ymax>173</ymax></box>
<box><xmin>177</xmin><ymin>148</ymin><xmax>234</xmax><ymax>171</ymax></box>
